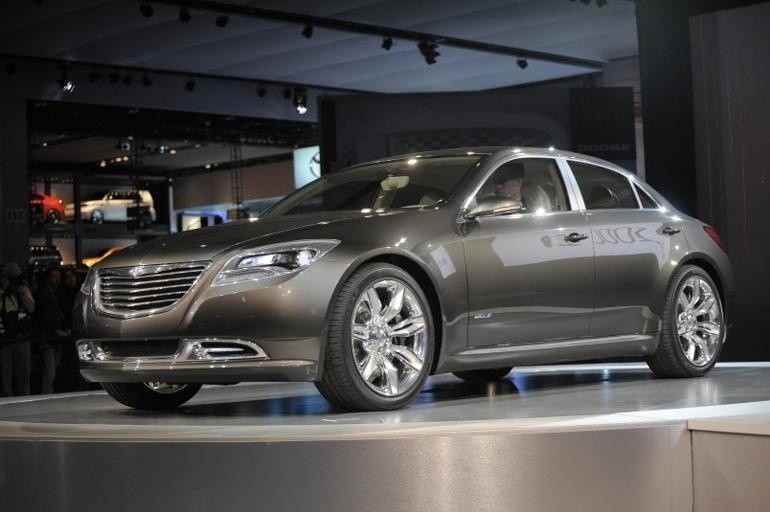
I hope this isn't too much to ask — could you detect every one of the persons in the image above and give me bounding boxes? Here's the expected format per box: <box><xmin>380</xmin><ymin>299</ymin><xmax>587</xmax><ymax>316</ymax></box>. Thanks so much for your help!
<box><xmin>1</xmin><ymin>259</ymin><xmax>36</xmax><ymax>397</ymax></box>
<box><xmin>490</xmin><ymin>163</ymin><xmax>527</xmax><ymax>205</ymax></box>
<box><xmin>58</xmin><ymin>270</ymin><xmax>85</xmax><ymax>392</ymax></box>
<box><xmin>32</xmin><ymin>265</ymin><xmax>73</xmax><ymax>394</ymax></box>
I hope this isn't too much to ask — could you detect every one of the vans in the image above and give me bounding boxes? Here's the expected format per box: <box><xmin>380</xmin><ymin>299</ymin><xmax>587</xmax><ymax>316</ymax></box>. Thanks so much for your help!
<box><xmin>28</xmin><ymin>244</ymin><xmax>63</xmax><ymax>267</ymax></box>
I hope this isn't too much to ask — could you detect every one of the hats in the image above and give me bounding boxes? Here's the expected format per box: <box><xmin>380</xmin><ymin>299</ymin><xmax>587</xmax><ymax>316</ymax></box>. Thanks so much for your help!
<box><xmin>2</xmin><ymin>261</ymin><xmax>23</xmax><ymax>275</ymax></box>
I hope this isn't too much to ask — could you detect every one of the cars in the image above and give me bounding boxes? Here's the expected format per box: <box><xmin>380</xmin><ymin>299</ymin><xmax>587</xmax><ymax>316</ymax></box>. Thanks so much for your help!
<box><xmin>67</xmin><ymin>142</ymin><xmax>740</xmax><ymax>416</ymax></box>
<box><xmin>29</xmin><ymin>191</ymin><xmax>64</xmax><ymax>223</ymax></box>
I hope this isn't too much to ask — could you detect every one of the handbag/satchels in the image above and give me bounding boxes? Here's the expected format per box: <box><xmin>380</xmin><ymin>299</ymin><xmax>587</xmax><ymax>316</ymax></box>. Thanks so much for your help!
<box><xmin>3</xmin><ymin>310</ymin><xmax>32</xmax><ymax>333</ymax></box>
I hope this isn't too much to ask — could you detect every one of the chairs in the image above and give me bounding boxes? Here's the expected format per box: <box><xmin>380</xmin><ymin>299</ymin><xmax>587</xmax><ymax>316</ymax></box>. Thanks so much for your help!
<box><xmin>586</xmin><ymin>185</ymin><xmax>621</xmax><ymax>209</ymax></box>
<box><xmin>519</xmin><ymin>183</ymin><xmax>551</xmax><ymax>213</ymax></box>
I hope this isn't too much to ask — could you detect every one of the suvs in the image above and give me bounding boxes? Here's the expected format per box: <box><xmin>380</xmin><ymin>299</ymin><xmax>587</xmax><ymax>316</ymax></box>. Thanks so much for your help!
<box><xmin>64</xmin><ymin>189</ymin><xmax>156</xmax><ymax>224</ymax></box>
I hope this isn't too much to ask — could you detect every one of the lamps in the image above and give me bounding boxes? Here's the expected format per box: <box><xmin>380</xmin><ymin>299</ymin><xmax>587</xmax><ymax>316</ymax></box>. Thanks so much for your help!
<box><xmin>125</xmin><ymin>1</ymin><xmax>442</xmax><ymax>66</ymax></box>
